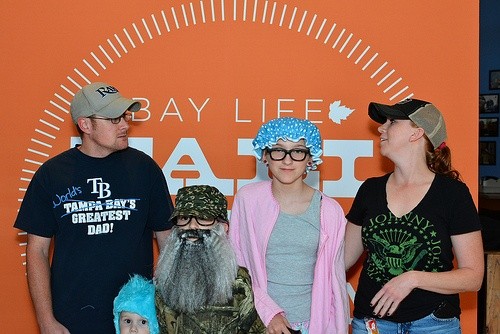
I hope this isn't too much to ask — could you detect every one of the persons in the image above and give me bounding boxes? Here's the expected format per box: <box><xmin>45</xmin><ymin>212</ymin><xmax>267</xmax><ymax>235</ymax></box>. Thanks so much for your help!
<box><xmin>343</xmin><ymin>99</ymin><xmax>486</xmax><ymax>334</ymax></box>
<box><xmin>13</xmin><ymin>81</ymin><xmax>177</xmax><ymax>334</ymax></box>
<box><xmin>112</xmin><ymin>274</ymin><xmax>160</xmax><ymax>334</ymax></box>
<box><xmin>154</xmin><ymin>184</ymin><xmax>267</xmax><ymax>334</ymax></box>
<box><xmin>228</xmin><ymin>117</ymin><xmax>350</xmax><ymax>334</ymax></box>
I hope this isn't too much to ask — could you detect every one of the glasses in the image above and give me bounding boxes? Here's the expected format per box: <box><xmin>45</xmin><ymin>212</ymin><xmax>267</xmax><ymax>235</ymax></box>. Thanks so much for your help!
<box><xmin>89</xmin><ymin>113</ymin><xmax>132</xmax><ymax>124</ymax></box>
<box><xmin>265</xmin><ymin>147</ymin><xmax>309</xmax><ymax>162</ymax></box>
<box><xmin>175</xmin><ymin>216</ymin><xmax>216</xmax><ymax>226</ymax></box>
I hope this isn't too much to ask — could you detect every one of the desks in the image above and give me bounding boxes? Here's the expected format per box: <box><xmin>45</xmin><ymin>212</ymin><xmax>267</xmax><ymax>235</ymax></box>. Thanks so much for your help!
<box><xmin>478</xmin><ymin>194</ymin><xmax>500</xmax><ymax>212</ymax></box>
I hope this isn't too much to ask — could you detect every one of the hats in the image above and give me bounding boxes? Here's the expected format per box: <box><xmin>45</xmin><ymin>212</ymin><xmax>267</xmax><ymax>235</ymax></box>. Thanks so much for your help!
<box><xmin>168</xmin><ymin>184</ymin><xmax>229</xmax><ymax>226</ymax></box>
<box><xmin>252</xmin><ymin>117</ymin><xmax>324</xmax><ymax>174</ymax></box>
<box><xmin>70</xmin><ymin>82</ymin><xmax>141</xmax><ymax>126</ymax></box>
<box><xmin>367</xmin><ymin>98</ymin><xmax>446</xmax><ymax>153</ymax></box>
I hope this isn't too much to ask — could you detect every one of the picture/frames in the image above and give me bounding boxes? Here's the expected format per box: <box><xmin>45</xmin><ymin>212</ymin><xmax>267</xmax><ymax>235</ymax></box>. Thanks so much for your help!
<box><xmin>479</xmin><ymin>140</ymin><xmax>496</xmax><ymax>166</ymax></box>
<box><xmin>488</xmin><ymin>70</ymin><xmax>500</xmax><ymax>91</ymax></box>
<box><xmin>479</xmin><ymin>93</ymin><xmax>500</xmax><ymax>114</ymax></box>
<box><xmin>479</xmin><ymin>116</ymin><xmax>500</xmax><ymax>137</ymax></box>
